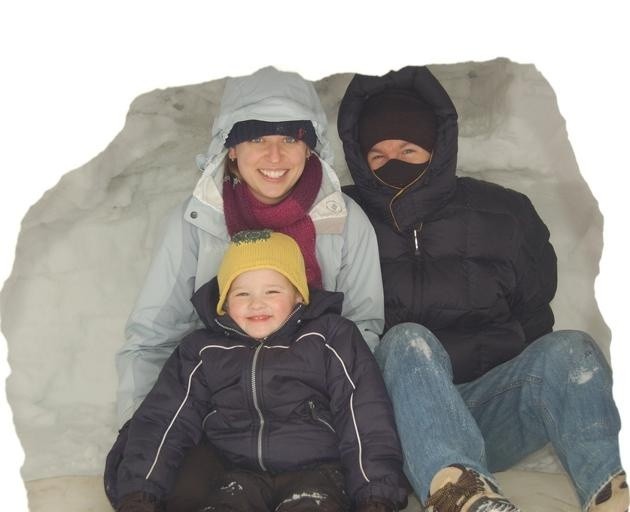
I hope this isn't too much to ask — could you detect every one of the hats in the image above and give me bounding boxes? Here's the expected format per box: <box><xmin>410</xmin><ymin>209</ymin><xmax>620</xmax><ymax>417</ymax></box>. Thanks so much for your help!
<box><xmin>225</xmin><ymin>120</ymin><xmax>318</xmax><ymax>148</ymax></box>
<box><xmin>216</xmin><ymin>229</ymin><xmax>309</xmax><ymax>316</ymax></box>
<box><xmin>357</xmin><ymin>90</ymin><xmax>439</xmax><ymax>163</ymax></box>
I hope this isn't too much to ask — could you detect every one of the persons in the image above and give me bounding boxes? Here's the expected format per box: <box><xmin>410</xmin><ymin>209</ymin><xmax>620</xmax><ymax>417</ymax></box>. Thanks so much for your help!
<box><xmin>103</xmin><ymin>229</ymin><xmax>414</xmax><ymax>512</ymax></box>
<box><xmin>340</xmin><ymin>66</ymin><xmax>629</xmax><ymax>512</ymax></box>
<box><xmin>114</xmin><ymin>66</ymin><xmax>385</xmax><ymax>443</ymax></box>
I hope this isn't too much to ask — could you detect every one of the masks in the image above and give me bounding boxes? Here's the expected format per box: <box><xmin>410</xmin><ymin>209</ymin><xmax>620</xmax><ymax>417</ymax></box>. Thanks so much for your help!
<box><xmin>375</xmin><ymin>158</ymin><xmax>430</xmax><ymax>188</ymax></box>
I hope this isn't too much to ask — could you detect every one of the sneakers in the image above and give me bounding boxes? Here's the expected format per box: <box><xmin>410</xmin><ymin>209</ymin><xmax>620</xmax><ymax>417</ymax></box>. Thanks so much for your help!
<box><xmin>423</xmin><ymin>464</ymin><xmax>520</xmax><ymax>511</ymax></box>
